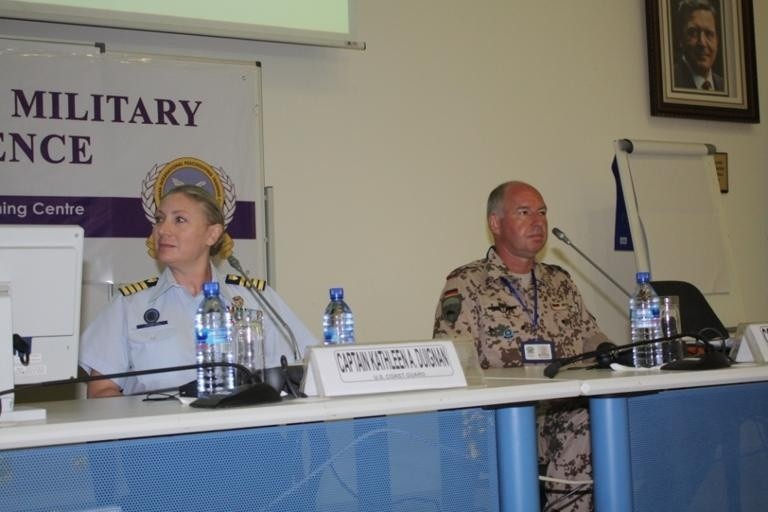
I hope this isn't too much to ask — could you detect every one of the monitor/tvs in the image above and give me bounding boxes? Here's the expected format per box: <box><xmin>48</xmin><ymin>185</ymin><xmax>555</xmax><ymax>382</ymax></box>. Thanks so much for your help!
<box><xmin>0</xmin><ymin>221</ymin><xmax>84</xmax><ymax>425</ymax></box>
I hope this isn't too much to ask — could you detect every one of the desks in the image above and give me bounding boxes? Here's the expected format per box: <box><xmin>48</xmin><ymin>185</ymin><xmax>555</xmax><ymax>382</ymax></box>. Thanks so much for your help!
<box><xmin>0</xmin><ymin>361</ymin><xmax>580</xmax><ymax>512</ymax></box>
<box><xmin>468</xmin><ymin>361</ymin><xmax>768</xmax><ymax>512</ymax></box>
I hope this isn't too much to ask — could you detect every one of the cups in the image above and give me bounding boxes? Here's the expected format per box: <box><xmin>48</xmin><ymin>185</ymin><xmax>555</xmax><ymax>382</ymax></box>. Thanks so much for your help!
<box><xmin>229</xmin><ymin>307</ymin><xmax>265</xmax><ymax>384</ymax></box>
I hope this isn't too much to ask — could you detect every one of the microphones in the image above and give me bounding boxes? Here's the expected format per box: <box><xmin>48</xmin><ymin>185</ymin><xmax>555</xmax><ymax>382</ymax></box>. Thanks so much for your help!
<box><xmin>225</xmin><ymin>252</ymin><xmax>303</xmax><ymax>361</ymax></box>
<box><xmin>2</xmin><ymin>359</ymin><xmax>280</xmax><ymax>407</ymax></box>
<box><xmin>549</xmin><ymin>225</ymin><xmax>635</xmax><ymax>302</ymax></box>
<box><xmin>543</xmin><ymin>330</ymin><xmax>732</xmax><ymax>379</ymax></box>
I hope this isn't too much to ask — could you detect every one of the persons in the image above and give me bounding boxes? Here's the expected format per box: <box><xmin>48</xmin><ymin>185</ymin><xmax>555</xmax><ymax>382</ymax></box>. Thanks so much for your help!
<box><xmin>79</xmin><ymin>185</ymin><xmax>321</xmax><ymax>511</ymax></box>
<box><xmin>674</xmin><ymin>1</ymin><xmax>725</xmax><ymax>91</ymax></box>
<box><xmin>433</xmin><ymin>181</ymin><xmax>610</xmax><ymax>512</ymax></box>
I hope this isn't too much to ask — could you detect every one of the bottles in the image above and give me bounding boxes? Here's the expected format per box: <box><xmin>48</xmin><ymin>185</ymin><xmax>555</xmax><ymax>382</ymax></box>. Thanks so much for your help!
<box><xmin>628</xmin><ymin>272</ymin><xmax>664</xmax><ymax>368</ymax></box>
<box><xmin>659</xmin><ymin>295</ymin><xmax>683</xmax><ymax>362</ymax></box>
<box><xmin>321</xmin><ymin>287</ymin><xmax>356</xmax><ymax>346</ymax></box>
<box><xmin>192</xmin><ymin>282</ymin><xmax>235</xmax><ymax>399</ymax></box>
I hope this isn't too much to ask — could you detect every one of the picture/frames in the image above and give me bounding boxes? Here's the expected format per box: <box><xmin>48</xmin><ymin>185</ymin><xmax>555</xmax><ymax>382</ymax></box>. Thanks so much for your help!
<box><xmin>646</xmin><ymin>0</ymin><xmax>760</xmax><ymax>123</ymax></box>
